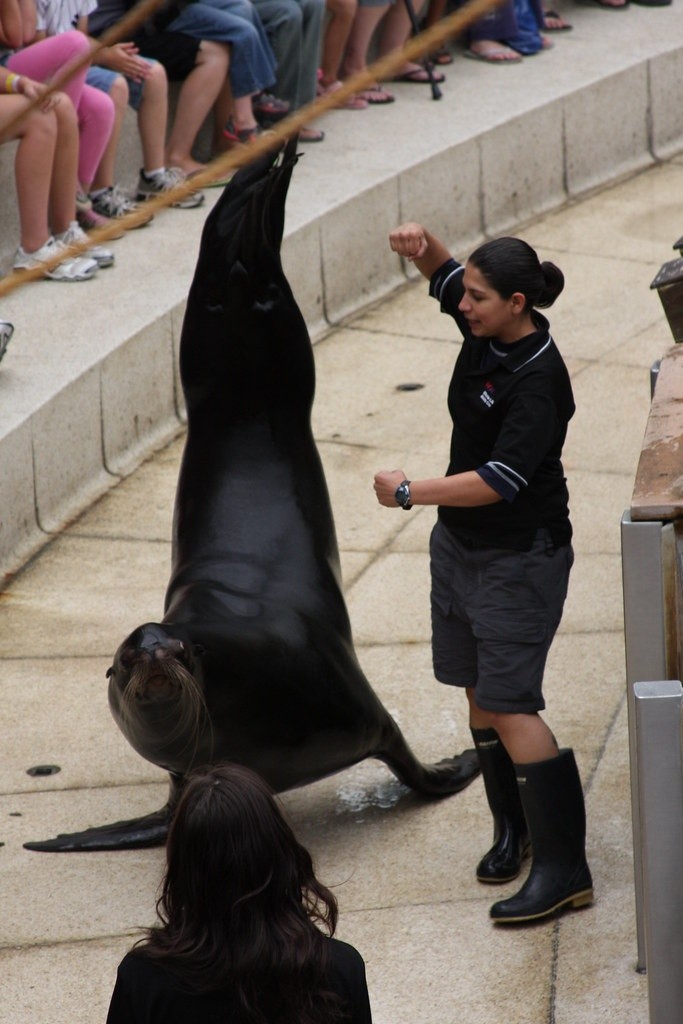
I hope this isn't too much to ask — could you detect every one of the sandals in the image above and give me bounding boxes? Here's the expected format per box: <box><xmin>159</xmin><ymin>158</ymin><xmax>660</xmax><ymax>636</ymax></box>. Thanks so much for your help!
<box><xmin>316</xmin><ymin>79</ymin><xmax>368</xmax><ymax>110</ymax></box>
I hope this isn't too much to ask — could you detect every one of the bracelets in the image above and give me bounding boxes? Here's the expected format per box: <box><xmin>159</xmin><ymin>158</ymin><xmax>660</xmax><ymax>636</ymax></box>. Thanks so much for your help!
<box><xmin>6</xmin><ymin>74</ymin><xmax>16</xmax><ymax>93</ymax></box>
<box><xmin>13</xmin><ymin>76</ymin><xmax>20</xmax><ymax>93</ymax></box>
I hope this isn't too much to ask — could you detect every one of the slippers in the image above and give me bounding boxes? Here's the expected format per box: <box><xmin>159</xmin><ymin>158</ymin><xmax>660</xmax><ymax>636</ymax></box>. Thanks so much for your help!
<box><xmin>299</xmin><ymin>127</ymin><xmax>324</xmax><ymax>142</ymax></box>
<box><xmin>183</xmin><ymin>166</ymin><xmax>234</xmax><ymax>187</ymax></box>
<box><xmin>540</xmin><ymin>9</ymin><xmax>573</xmax><ymax>33</ymax></box>
<box><xmin>597</xmin><ymin>0</ymin><xmax>630</xmax><ymax>10</ymax></box>
<box><xmin>393</xmin><ymin>62</ymin><xmax>445</xmax><ymax>82</ymax></box>
<box><xmin>463</xmin><ymin>38</ymin><xmax>523</xmax><ymax>65</ymax></box>
<box><xmin>432</xmin><ymin>47</ymin><xmax>454</xmax><ymax>65</ymax></box>
<box><xmin>353</xmin><ymin>85</ymin><xmax>395</xmax><ymax>105</ymax></box>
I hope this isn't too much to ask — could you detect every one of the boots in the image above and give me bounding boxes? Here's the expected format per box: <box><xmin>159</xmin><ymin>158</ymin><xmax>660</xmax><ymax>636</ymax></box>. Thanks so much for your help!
<box><xmin>487</xmin><ymin>748</ymin><xmax>593</xmax><ymax>923</ymax></box>
<box><xmin>467</xmin><ymin>720</ymin><xmax>532</xmax><ymax>884</ymax></box>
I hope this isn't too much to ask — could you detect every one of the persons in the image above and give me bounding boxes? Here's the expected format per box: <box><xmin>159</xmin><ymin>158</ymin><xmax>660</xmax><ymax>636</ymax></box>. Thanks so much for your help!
<box><xmin>372</xmin><ymin>222</ymin><xmax>594</xmax><ymax>923</ymax></box>
<box><xmin>0</xmin><ymin>0</ymin><xmax>630</xmax><ymax>280</ymax></box>
<box><xmin>107</xmin><ymin>766</ymin><xmax>372</xmax><ymax>1023</ymax></box>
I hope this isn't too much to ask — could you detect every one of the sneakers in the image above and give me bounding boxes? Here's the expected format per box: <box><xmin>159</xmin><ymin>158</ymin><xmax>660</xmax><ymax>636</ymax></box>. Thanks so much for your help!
<box><xmin>134</xmin><ymin>165</ymin><xmax>204</xmax><ymax>208</ymax></box>
<box><xmin>251</xmin><ymin>94</ymin><xmax>289</xmax><ymax>112</ymax></box>
<box><xmin>87</xmin><ymin>182</ymin><xmax>155</xmax><ymax>229</ymax></box>
<box><xmin>73</xmin><ymin>199</ymin><xmax>126</xmax><ymax>239</ymax></box>
<box><xmin>12</xmin><ymin>236</ymin><xmax>98</xmax><ymax>282</ymax></box>
<box><xmin>54</xmin><ymin>220</ymin><xmax>114</xmax><ymax>267</ymax></box>
<box><xmin>221</xmin><ymin>115</ymin><xmax>266</xmax><ymax>145</ymax></box>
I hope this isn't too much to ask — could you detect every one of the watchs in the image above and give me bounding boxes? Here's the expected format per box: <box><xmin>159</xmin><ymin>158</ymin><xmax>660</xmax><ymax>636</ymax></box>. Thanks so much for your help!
<box><xmin>395</xmin><ymin>480</ymin><xmax>413</xmax><ymax>510</ymax></box>
